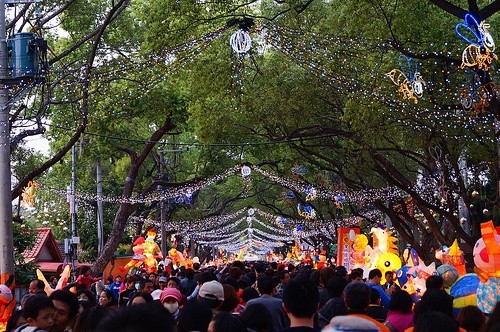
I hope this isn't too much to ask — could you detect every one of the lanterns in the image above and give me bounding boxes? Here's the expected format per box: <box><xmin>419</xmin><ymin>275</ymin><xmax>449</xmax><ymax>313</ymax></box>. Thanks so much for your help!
<box><xmin>115</xmin><ymin>228</ymin><xmax>258</xmax><ymax>274</ymax></box>
<box><xmin>346</xmin><ymin>223</ymin><xmax>471</xmax><ymax>316</ymax></box>
<box><xmin>260</xmin><ymin>242</ymin><xmax>338</xmax><ymax>268</ymax></box>
<box><xmin>472</xmin><ymin>220</ymin><xmax>500</xmax><ymax>316</ymax></box>
<box><xmin>1</xmin><ymin>284</ymin><xmax>18</xmax><ymax>332</ymax></box>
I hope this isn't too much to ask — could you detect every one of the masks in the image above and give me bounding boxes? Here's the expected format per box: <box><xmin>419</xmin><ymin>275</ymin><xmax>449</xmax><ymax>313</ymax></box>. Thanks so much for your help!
<box><xmin>163</xmin><ymin>301</ymin><xmax>179</xmax><ymax>313</ymax></box>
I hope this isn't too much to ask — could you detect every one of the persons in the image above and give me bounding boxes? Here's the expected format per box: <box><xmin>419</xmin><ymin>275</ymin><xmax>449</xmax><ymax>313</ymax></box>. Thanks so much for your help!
<box><xmin>175</xmin><ymin>302</ymin><xmax>213</xmax><ymax>332</ymax></box>
<box><xmin>13</xmin><ymin>293</ymin><xmax>55</xmax><ymax>331</ymax></box>
<box><xmin>95</xmin><ymin>305</ymin><xmax>171</xmax><ymax>332</ymax></box>
<box><xmin>323</xmin><ymin>280</ymin><xmax>391</xmax><ymax>332</ymax></box>
<box><xmin>280</xmin><ymin>278</ymin><xmax>319</xmax><ymax>331</ymax></box>
<box><xmin>44</xmin><ymin>289</ymin><xmax>79</xmax><ymax>332</ymax></box>
<box><xmin>20</xmin><ymin>260</ymin><xmax>500</xmax><ymax>320</ymax></box>
<box><xmin>207</xmin><ymin>312</ymin><xmax>247</xmax><ymax>332</ymax></box>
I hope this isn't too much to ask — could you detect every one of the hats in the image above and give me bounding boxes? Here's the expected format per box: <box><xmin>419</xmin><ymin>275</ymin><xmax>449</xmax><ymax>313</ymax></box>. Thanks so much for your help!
<box><xmin>198</xmin><ymin>280</ymin><xmax>224</xmax><ymax>300</ymax></box>
<box><xmin>159</xmin><ymin>288</ymin><xmax>182</xmax><ymax>303</ymax></box>
<box><xmin>151</xmin><ymin>289</ymin><xmax>164</xmax><ymax>301</ymax></box>
<box><xmin>159</xmin><ymin>276</ymin><xmax>167</xmax><ymax>283</ymax></box>
